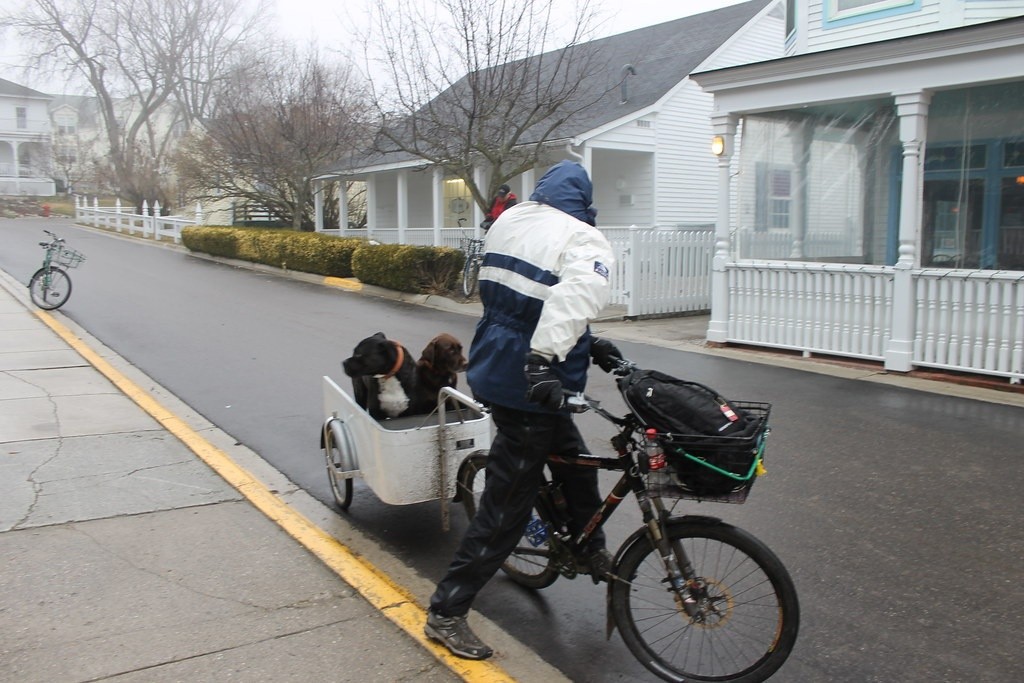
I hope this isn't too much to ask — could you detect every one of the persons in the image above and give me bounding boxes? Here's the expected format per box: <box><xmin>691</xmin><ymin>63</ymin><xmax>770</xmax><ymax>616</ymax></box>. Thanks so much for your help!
<box><xmin>482</xmin><ymin>184</ymin><xmax>516</xmax><ymax>232</ymax></box>
<box><xmin>425</xmin><ymin>163</ymin><xmax>625</xmax><ymax>659</ymax></box>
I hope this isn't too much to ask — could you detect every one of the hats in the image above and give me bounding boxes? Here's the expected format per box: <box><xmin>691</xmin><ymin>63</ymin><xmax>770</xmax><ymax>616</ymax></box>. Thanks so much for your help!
<box><xmin>498</xmin><ymin>183</ymin><xmax>512</xmax><ymax>194</ymax></box>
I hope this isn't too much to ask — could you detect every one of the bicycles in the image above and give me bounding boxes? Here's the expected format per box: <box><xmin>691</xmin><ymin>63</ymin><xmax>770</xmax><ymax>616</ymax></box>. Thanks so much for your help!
<box><xmin>451</xmin><ymin>353</ymin><xmax>802</xmax><ymax>683</ymax></box>
<box><xmin>456</xmin><ymin>216</ymin><xmax>490</xmax><ymax>298</ymax></box>
<box><xmin>26</xmin><ymin>228</ymin><xmax>84</xmax><ymax>312</ymax></box>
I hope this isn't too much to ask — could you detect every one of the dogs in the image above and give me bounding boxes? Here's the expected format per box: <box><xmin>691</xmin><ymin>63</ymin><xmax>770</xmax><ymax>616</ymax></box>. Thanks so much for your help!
<box><xmin>341</xmin><ymin>331</ymin><xmax>469</xmax><ymax>423</ymax></box>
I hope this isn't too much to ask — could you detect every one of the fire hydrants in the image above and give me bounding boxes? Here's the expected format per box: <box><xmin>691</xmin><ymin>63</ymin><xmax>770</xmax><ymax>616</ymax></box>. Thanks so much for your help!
<box><xmin>42</xmin><ymin>204</ymin><xmax>51</xmax><ymax>217</ymax></box>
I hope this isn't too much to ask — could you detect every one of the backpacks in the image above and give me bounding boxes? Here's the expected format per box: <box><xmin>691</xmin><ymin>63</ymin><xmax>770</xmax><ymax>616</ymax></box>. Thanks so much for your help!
<box><xmin>617</xmin><ymin>367</ymin><xmax>761</xmax><ymax>452</ymax></box>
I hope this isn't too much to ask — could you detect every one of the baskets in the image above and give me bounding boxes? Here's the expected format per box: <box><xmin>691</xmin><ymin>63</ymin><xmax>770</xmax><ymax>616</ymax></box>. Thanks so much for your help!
<box><xmin>638</xmin><ymin>401</ymin><xmax>774</xmax><ymax>504</ymax></box>
<box><xmin>459</xmin><ymin>238</ymin><xmax>480</xmax><ymax>251</ymax></box>
<box><xmin>54</xmin><ymin>243</ymin><xmax>86</xmax><ymax>270</ymax></box>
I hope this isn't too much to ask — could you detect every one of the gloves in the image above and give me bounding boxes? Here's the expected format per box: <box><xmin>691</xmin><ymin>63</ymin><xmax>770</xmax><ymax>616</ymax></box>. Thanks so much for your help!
<box><xmin>482</xmin><ymin>215</ymin><xmax>493</xmax><ymax>223</ymax></box>
<box><xmin>588</xmin><ymin>334</ymin><xmax>623</xmax><ymax>373</ymax></box>
<box><xmin>522</xmin><ymin>350</ymin><xmax>567</xmax><ymax>410</ymax></box>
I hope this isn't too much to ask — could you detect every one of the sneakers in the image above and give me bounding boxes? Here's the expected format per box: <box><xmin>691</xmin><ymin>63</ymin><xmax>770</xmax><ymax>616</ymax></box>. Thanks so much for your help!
<box><xmin>573</xmin><ymin>549</ymin><xmax>636</xmax><ymax>582</ymax></box>
<box><xmin>423</xmin><ymin>607</ymin><xmax>494</xmax><ymax>659</ymax></box>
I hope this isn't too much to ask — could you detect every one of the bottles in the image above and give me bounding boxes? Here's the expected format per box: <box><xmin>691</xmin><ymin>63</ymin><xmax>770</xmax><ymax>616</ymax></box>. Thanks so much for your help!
<box><xmin>644</xmin><ymin>429</ymin><xmax>671</xmax><ymax>489</ymax></box>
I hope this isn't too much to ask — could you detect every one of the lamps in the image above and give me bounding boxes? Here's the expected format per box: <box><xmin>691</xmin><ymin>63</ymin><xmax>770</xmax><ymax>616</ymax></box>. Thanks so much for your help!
<box><xmin>711</xmin><ymin>135</ymin><xmax>724</xmax><ymax>155</ymax></box>
